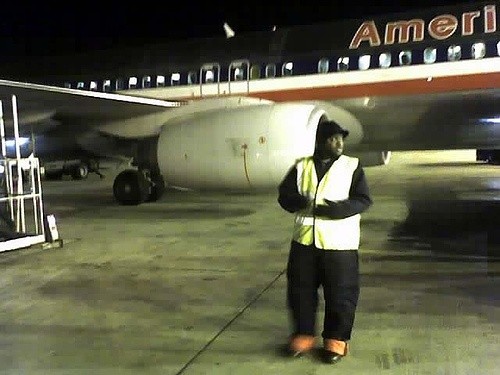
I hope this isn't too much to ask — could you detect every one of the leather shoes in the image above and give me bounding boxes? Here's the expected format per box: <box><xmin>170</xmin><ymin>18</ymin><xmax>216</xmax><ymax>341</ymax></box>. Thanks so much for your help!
<box><xmin>319</xmin><ymin>338</ymin><xmax>349</xmax><ymax>364</ymax></box>
<box><xmin>286</xmin><ymin>334</ymin><xmax>315</xmax><ymax>358</ymax></box>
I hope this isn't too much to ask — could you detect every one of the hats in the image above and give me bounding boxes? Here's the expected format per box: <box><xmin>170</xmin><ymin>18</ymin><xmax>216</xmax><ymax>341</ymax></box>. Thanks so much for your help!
<box><xmin>317</xmin><ymin>119</ymin><xmax>350</xmax><ymax>145</ymax></box>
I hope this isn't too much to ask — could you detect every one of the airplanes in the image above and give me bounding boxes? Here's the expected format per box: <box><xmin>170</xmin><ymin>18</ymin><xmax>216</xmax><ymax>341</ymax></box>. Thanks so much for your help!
<box><xmin>0</xmin><ymin>0</ymin><xmax>500</xmax><ymax>206</ymax></box>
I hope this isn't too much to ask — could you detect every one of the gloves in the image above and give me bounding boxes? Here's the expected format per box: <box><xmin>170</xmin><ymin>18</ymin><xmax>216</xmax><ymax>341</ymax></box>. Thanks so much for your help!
<box><xmin>287</xmin><ymin>192</ymin><xmax>308</xmax><ymax>214</ymax></box>
<box><xmin>312</xmin><ymin>199</ymin><xmax>349</xmax><ymax>219</ymax></box>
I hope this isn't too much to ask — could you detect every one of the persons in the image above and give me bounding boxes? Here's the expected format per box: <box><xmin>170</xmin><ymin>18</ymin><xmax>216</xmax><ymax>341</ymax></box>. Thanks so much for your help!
<box><xmin>278</xmin><ymin>120</ymin><xmax>373</xmax><ymax>364</ymax></box>
